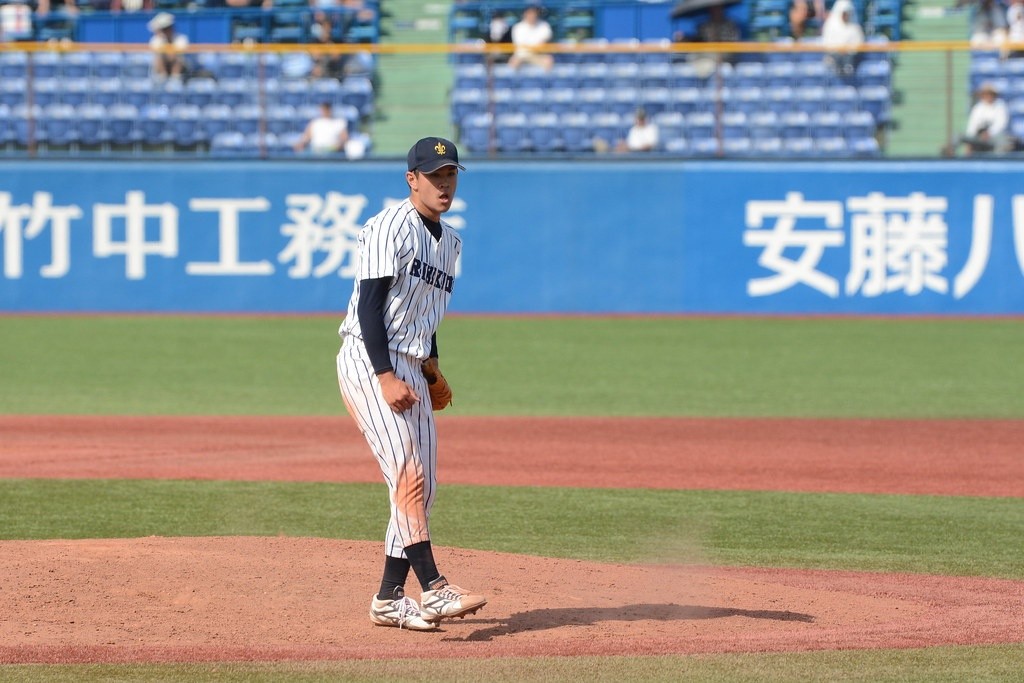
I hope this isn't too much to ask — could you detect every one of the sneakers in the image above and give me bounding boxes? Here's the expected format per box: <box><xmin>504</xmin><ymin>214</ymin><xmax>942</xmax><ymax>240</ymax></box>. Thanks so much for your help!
<box><xmin>420</xmin><ymin>575</ymin><xmax>487</xmax><ymax>621</ymax></box>
<box><xmin>368</xmin><ymin>592</ymin><xmax>437</xmax><ymax>629</ymax></box>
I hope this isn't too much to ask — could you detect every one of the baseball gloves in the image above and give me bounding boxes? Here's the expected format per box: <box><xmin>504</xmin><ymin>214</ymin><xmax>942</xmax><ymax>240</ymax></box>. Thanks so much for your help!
<box><xmin>421</xmin><ymin>357</ymin><xmax>453</xmax><ymax>412</ymax></box>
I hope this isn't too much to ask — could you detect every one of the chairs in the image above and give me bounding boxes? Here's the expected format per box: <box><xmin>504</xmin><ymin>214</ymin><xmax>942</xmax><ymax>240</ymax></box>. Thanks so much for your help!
<box><xmin>0</xmin><ymin>1</ymin><xmax>1024</xmax><ymax>164</ymax></box>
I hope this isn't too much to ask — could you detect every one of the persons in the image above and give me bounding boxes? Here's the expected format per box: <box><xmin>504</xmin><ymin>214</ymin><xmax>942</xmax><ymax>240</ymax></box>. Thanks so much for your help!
<box><xmin>955</xmin><ymin>84</ymin><xmax>1014</xmax><ymax>158</ymax></box>
<box><xmin>337</xmin><ymin>136</ymin><xmax>486</xmax><ymax>631</ymax></box>
<box><xmin>673</xmin><ymin>6</ymin><xmax>740</xmax><ymax>43</ymax></box>
<box><xmin>963</xmin><ymin>0</ymin><xmax>1024</xmax><ymax>56</ymax></box>
<box><xmin>293</xmin><ymin>100</ymin><xmax>349</xmax><ymax>152</ymax></box>
<box><xmin>479</xmin><ymin>6</ymin><xmax>555</xmax><ymax>73</ymax></box>
<box><xmin>789</xmin><ymin>0</ymin><xmax>864</xmax><ymax>75</ymax></box>
<box><xmin>0</xmin><ymin>0</ymin><xmax>375</xmax><ymax>83</ymax></box>
<box><xmin>592</xmin><ymin>103</ymin><xmax>659</xmax><ymax>153</ymax></box>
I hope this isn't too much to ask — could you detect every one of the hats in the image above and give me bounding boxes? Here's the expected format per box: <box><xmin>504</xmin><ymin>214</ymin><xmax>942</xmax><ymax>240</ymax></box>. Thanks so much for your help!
<box><xmin>146</xmin><ymin>12</ymin><xmax>176</xmax><ymax>33</ymax></box>
<box><xmin>977</xmin><ymin>80</ymin><xmax>999</xmax><ymax>94</ymax></box>
<box><xmin>407</xmin><ymin>137</ymin><xmax>467</xmax><ymax>174</ymax></box>
